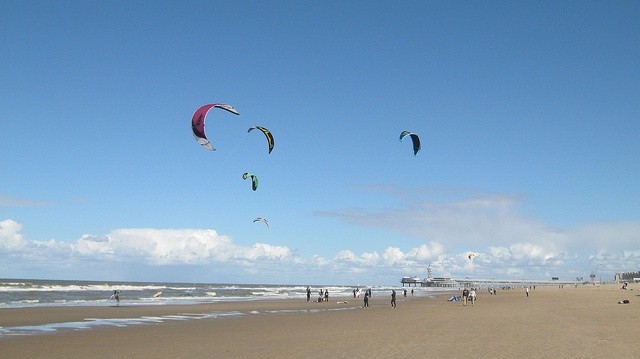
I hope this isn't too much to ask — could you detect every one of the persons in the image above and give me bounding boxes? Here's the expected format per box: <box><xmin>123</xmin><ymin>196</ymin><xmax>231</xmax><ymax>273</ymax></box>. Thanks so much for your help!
<box><xmin>411</xmin><ymin>289</ymin><xmax>415</xmax><ymax>296</ymax></box>
<box><xmin>463</xmin><ymin>287</ymin><xmax>469</xmax><ymax>306</ymax></box>
<box><xmin>403</xmin><ymin>289</ymin><xmax>407</xmax><ymax>296</ymax></box>
<box><xmin>356</xmin><ymin>287</ymin><xmax>359</xmax><ymax>292</ymax></box>
<box><xmin>476</xmin><ymin>284</ymin><xmax>521</xmax><ymax>296</ymax></box>
<box><xmin>468</xmin><ymin>287</ymin><xmax>476</xmax><ymax>306</ymax></box>
<box><xmin>325</xmin><ymin>289</ymin><xmax>329</xmax><ymax>302</ymax></box>
<box><xmin>363</xmin><ymin>290</ymin><xmax>369</xmax><ymax>308</ymax></box>
<box><xmin>305</xmin><ymin>285</ymin><xmax>311</xmax><ymax>303</ymax></box>
<box><xmin>353</xmin><ymin>288</ymin><xmax>356</xmax><ymax>298</ymax></box>
<box><xmin>113</xmin><ymin>290</ymin><xmax>120</xmax><ymax>303</ymax></box>
<box><xmin>319</xmin><ymin>289</ymin><xmax>324</xmax><ymax>301</ymax></box>
<box><xmin>390</xmin><ymin>290</ymin><xmax>397</xmax><ymax>308</ymax></box>
<box><xmin>526</xmin><ymin>286</ymin><xmax>529</xmax><ymax>296</ymax></box>
